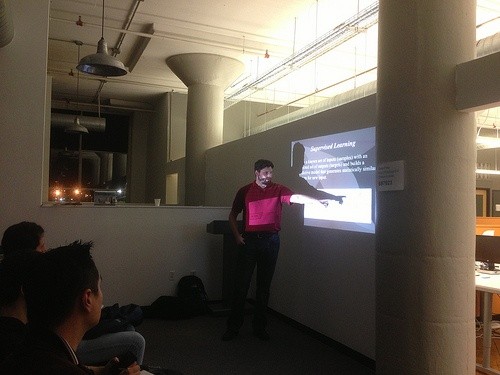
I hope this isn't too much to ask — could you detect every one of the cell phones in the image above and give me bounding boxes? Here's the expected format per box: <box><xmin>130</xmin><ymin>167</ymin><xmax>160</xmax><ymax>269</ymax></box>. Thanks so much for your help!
<box><xmin>111</xmin><ymin>350</ymin><xmax>138</xmax><ymax>375</ymax></box>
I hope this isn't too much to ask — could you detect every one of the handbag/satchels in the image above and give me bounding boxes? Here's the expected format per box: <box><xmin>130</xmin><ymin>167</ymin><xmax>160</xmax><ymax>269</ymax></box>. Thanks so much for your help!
<box><xmin>96</xmin><ymin>303</ymin><xmax>129</xmax><ymax>333</ymax></box>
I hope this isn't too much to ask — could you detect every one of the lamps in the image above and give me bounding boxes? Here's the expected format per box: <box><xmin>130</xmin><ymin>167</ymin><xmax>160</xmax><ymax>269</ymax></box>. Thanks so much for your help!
<box><xmin>76</xmin><ymin>0</ymin><xmax>128</xmax><ymax>77</ymax></box>
<box><xmin>64</xmin><ymin>41</ymin><xmax>89</xmax><ymax>135</ymax></box>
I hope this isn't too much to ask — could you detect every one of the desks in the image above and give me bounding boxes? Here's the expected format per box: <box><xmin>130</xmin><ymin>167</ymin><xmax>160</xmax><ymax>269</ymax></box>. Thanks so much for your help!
<box><xmin>474</xmin><ymin>261</ymin><xmax>500</xmax><ymax>375</ymax></box>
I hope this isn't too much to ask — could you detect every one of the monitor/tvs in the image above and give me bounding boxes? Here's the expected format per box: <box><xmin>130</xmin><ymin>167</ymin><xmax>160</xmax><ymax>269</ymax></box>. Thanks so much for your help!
<box><xmin>475</xmin><ymin>235</ymin><xmax>500</xmax><ymax>271</ymax></box>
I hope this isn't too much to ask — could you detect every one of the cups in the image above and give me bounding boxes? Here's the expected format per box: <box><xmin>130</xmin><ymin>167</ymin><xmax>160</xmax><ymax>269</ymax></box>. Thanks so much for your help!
<box><xmin>154</xmin><ymin>198</ymin><xmax>161</xmax><ymax>206</ymax></box>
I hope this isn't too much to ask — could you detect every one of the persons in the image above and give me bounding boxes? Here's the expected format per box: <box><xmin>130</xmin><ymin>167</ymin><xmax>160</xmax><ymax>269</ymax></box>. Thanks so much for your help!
<box><xmin>0</xmin><ymin>221</ymin><xmax>146</xmax><ymax>375</ymax></box>
<box><xmin>221</xmin><ymin>159</ymin><xmax>329</xmax><ymax>342</ymax></box>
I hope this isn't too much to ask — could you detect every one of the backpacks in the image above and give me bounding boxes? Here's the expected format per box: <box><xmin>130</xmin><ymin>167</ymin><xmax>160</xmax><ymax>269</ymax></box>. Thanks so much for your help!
<box><xmin>178</xmin><ymin>274</ymin><xmax>211</xmax><ymax>316</ymax></box>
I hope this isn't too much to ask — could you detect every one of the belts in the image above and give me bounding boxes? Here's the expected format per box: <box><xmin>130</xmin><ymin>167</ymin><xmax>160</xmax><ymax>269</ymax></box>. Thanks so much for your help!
<box><xmin>242</xmin><ymin>231</ymin><xmax>272</xmax><ymax>239</ymax></box>
